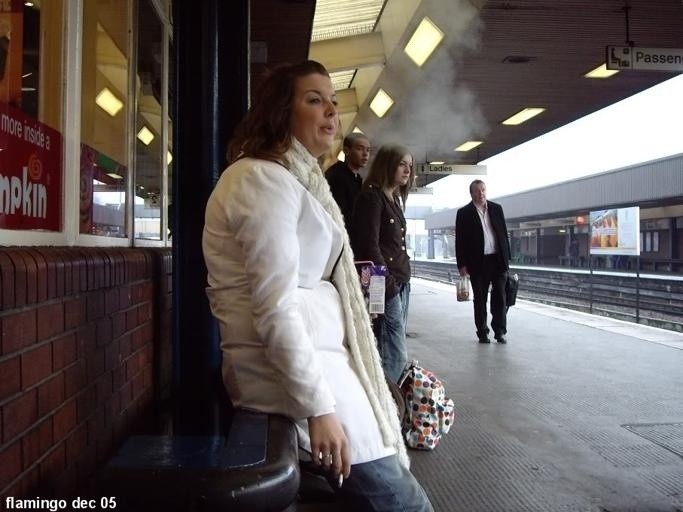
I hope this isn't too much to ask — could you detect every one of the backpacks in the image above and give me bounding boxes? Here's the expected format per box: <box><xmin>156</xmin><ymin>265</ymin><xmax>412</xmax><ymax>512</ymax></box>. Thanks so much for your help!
<box><xmin>396</xmin><ymin>360</ymin><xmax>455</xmax><ymax>451</ymax></box>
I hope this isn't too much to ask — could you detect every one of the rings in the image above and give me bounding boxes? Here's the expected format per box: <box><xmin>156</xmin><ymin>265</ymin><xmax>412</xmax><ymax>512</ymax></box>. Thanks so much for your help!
<box><xmin>322</xmin><ymin>453</ymin><xmax>332</xmax><ymax>460</ymax></box>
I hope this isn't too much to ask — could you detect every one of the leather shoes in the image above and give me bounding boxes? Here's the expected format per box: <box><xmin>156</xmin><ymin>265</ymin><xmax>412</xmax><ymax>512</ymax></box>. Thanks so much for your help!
<box><xmin>479</xmin><ymin>333</ymin><xmax>507</xmax><ymax>343</ymax></box>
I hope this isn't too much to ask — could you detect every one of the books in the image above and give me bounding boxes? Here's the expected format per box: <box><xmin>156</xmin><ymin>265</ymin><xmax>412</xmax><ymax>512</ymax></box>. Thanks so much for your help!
<box><xmin>355</xmin><ymin>261</ymin><xmax>389</xmax><ymax>318</ymax></box>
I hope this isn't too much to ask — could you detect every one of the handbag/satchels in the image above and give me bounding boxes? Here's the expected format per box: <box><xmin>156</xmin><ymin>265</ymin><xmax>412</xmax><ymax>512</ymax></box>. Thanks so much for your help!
<box><xmin>352</xmin><ymin>261</ymin><xmax>400</xmax><ymax>307</ymax></box>
<box><xmin>505</xmin><ymin>271</ymin><xmax>518</xmax><ymax>306</ymax></box>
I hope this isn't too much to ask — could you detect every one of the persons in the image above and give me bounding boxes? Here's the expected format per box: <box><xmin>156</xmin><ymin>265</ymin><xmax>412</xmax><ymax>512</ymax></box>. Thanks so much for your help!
<box><xmin>200</xmin><ymin>56</ymin><xmax>440</xmax><ymax>512</ymax></box>
<box><xmin>453</xmin><ymin>178</ymin><xmax>511</xmax><ymax>344</ymax></box>
<box><xmin>321</xmin><ymin>132</ymin><xmax>371</xmax><ymax>230</ymax></box>
<box><xmin>342</xmin><ymin>142</ymin><xmax>415</xmax><ymax>386</ymax></box>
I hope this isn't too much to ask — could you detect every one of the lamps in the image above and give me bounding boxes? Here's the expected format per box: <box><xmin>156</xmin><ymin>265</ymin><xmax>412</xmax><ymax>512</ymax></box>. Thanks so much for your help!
<box><xmin>137</xmin><ymin>125</ymin><xmax>155</xmax><ymax>146</ymax></box>
<box><xmin>403</xmin><ymin>15</ymin><xmax>445</xmax><ymax>67</ymax></box>
<box><xmin>369</xmin><ymin>88</ymin><xmax>394</xmax><ymax>119</ymax></box>
<box><xmin>95</xmin><ymin>86</ymin><xmax>124</xmax><ymax>117</ymax></box>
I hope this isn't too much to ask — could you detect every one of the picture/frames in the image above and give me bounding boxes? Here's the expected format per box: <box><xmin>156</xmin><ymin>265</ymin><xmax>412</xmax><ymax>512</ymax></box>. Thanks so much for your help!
<box><xmin>0</xmin><ymin>5</ymin><xmax>41</xmax><ymax>120</ymax></box>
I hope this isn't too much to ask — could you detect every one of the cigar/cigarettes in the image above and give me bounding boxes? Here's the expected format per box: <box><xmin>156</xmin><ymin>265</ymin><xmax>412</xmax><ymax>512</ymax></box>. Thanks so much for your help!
<box><xmin>337</xmin><ymin>473</ymin><xmax>344</xmax><ymax>487</ymax></box>
